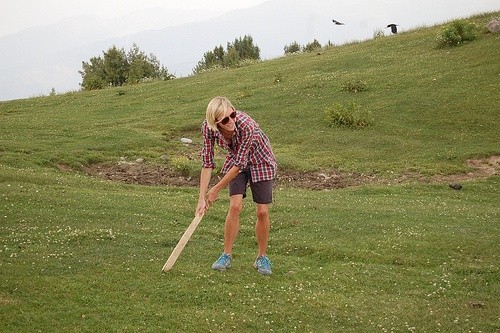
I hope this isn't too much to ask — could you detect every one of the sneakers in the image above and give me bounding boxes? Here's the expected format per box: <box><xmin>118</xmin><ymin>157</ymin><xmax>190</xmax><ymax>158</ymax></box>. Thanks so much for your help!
<box><xmin>211</xmin><ymin>253</ymin><xmax>233</xmax><ymax>270</ymax></box>
<box><xmin>253</xmin><ymin>255</ymin><xmax>273</xmax><ymax>276</ymax></box>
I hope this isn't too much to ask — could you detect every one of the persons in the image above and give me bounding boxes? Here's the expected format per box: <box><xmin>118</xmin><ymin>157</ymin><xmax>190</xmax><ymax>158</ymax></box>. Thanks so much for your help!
<box><xmin>195</xmin><ymin>96</ymin><xmax>277</xmax><ymax>274</ymax></box>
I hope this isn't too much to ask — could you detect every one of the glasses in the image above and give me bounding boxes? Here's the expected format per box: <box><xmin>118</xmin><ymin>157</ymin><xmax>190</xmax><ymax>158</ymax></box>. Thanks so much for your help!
<box><xmin>215</xmin><ymin>110</ymin><xmax>237</xmax><ymax>125</ymax></box>
